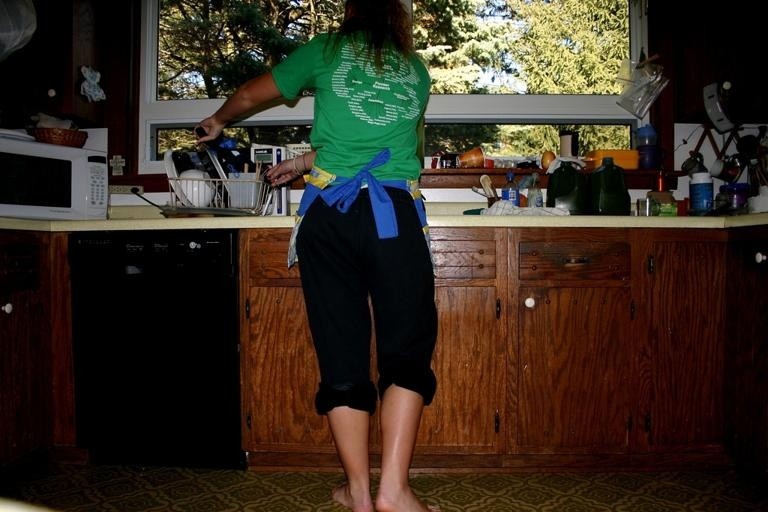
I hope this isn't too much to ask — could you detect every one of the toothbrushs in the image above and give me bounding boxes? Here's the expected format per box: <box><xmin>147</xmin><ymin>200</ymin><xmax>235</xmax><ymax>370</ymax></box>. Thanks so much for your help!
<box><xmin>470</xmin><ymin>185</ymin><xmax>486</xmax><ymax>197</ymax></box>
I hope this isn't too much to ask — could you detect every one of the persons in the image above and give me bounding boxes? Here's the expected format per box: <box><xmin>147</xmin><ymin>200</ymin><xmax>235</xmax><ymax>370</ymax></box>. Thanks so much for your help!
<box><xmin>190</xmin><ymin>0</ymin><xmax>439</xmax><ymax>511</ymax></box>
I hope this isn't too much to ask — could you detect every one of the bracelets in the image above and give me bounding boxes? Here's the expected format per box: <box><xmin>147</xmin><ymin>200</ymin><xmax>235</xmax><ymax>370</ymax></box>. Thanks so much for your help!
<box><xmin>291</xmin><ymin>156</ymin><xmax>305</xmax><ymax>176</ymax></box>
<box><xmin>302</xmin><ymin>153</ymin><xmax>308</xmax><ymax>172</ymax></box>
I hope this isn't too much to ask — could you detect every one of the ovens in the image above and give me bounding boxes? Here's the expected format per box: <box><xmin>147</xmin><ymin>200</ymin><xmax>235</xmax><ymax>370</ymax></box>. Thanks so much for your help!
<box><xmin>67</xmin><ymin>231</ymin><xmax>239</xmax><ymax>470</ymax></box>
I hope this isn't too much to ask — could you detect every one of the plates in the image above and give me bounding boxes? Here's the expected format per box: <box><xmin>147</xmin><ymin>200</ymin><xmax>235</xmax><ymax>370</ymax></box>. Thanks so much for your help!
<box><xmin>163</xmin><ymin>151</ymin><xmax>193</xmax><ymax>207</ymax></box>
<box><xmin>195</xmin><ymin>126</ymin><xmax>234</xmax><ymax>195</ymax></box>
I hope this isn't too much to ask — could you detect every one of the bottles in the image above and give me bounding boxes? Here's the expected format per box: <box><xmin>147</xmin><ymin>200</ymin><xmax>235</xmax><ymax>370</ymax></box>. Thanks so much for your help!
<box><xmin>689</xmin><ymin>172</ymin><xmax>713</xmax><ymax>217</ymax></box>
<box><xmin>634</xmin><ymin>125</ymin><xmax>657</xmax><ymax>171</ymax></box>
<box><xmin>503</xmin><ymin>174</ymin><xmax>519</xmax><ymax>207</ymax></box>
<box><xmin>528</xmin><ymin>170</ymin><xmax>544</xmax><ymax>208</ymax></box>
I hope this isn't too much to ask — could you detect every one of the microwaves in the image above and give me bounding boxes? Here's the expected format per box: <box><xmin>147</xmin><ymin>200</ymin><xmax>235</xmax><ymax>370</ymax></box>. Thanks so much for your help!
<box><xmin>1</xmin><ymin>137</ymin><xmax>109</xmax><ymax>225</ymax></box>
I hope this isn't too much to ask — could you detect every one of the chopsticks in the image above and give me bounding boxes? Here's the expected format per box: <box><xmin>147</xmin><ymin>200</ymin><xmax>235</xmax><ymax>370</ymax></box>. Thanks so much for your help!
<box><xmin>254</xmin><ymin>158</ymin><xmax>261</xmax><ymax>183</ymax></box>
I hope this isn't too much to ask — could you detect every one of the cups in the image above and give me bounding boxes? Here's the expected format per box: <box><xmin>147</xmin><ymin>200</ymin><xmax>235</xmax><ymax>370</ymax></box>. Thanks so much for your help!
<box><xmin>682</xmin><ymin>151</ymin><xmax>708</xmax><ymax>179</ymax></box>
<box><xmin>714</xmin><ymin>159</ymin><xmax>744</xmax><ymax>185</ymax></box>
<box><xmin>456</xmin><ymin>147</ymin><xmax>485</xmax><ymax>169</ymax></box>
<box><xmin>559</xmin><ymin>130</ymin><xmax>578</xmax><ymax>159</ymax></box>
<box><xmin>638</xmin><ymin>197</ymin><xmax>661</xmax><ymax>217</ymax></box>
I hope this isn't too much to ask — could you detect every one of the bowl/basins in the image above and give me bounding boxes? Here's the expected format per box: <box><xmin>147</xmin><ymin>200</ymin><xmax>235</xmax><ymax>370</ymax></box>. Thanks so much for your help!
<box><xmin>180</xmin><ymin>170</ymin><xmax>217</xmax><ymax>209</ymax></box>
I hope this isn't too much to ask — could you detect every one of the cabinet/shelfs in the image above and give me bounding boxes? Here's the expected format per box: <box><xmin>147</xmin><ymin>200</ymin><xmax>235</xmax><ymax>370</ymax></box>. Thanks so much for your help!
<box><xmin>0</xmin><ymin>230</ymin><xmax>77</xmax><ymax>493</ymax></box>
<box><xmin>236</xmin><ymin>228</ymin><xmax>728</xmax><ymax>476</ymax></box>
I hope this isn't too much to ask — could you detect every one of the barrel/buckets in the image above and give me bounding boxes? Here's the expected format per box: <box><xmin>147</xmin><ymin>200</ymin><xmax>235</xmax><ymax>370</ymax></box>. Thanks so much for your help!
<box><xmin>582</xmin><ymin>156</ymin><xmax>631</xmax><ymax>215</ymax></box>
<box><xmin>546</xmin><ymin>162</ymin><xmax>584</xmax><ymax>214</ymax></box>
